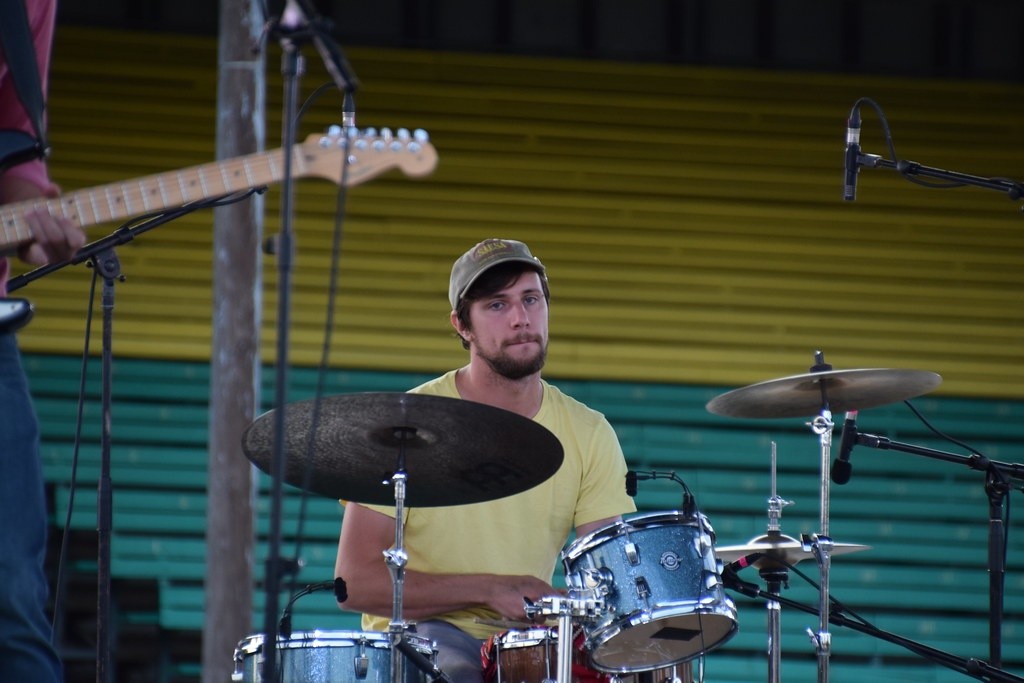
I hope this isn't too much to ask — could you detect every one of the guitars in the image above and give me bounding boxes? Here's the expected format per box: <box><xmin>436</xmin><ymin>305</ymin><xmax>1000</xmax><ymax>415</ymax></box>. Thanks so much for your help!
<box><xmin>0</xmin><ymin>128</ymin><xmax>438</xmax><ymax>337</ymax></box>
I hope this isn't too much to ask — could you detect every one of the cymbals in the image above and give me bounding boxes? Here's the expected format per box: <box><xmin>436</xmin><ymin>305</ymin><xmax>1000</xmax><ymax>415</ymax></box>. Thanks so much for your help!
<box><xmin>703</xmin><ymin>367</ymin><xmax>942</xmax><ymax>421</ymax></box>
<box><xmin>717</xmin><ymin>535</ymin><xmax>869</xmax><ymax>567</ymax></box>
<box><xmin>241</xmin><ymin>392</ymin><xmax>564</xmax><ymax>508</ymax></box>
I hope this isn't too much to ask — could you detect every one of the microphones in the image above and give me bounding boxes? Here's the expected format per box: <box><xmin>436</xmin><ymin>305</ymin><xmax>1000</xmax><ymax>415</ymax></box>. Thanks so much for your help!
<box><xmin>829</xmin><ymin>410</ymin><xmax>856</xmax><ymax>485</ymax></box>
<box><xmin>845</xmin><ymin>108</ymin><xmax>862</xmax><ymax>199</ymax></box>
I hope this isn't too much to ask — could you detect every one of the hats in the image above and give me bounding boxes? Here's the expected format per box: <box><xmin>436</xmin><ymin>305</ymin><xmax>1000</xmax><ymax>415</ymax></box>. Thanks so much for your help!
<box><xmin>448</xmin><ymin>240</ymin><xmax>548</xmax><ymax>312</ymax></box>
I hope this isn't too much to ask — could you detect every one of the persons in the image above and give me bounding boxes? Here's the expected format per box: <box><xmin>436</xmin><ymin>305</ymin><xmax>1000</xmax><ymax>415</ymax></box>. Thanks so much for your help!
<box><xmin>0</xmin><ymin>0</ymin><xmax>90</xmax><ymax>682</ymax></box>
<box><xmin>329</xmin><ymin>236</ymin><xmax>682</xmax><ymax>683</ymax></box>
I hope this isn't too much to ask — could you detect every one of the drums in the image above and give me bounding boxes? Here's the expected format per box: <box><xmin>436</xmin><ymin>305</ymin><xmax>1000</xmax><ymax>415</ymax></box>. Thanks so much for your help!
<box><xmin>561</xmin><ymin>511</ymin><xmax>740</xmax><ymax>675</ymax></box>
<box><xmin>234</xmin><ymin>628</ymin><xmax>435</xmax><ymax>683</ymax></box>
<box><xmin>484</xmin><ymin>631</ymin><xmax>691</xmax><ymax>681</ymax></box>
